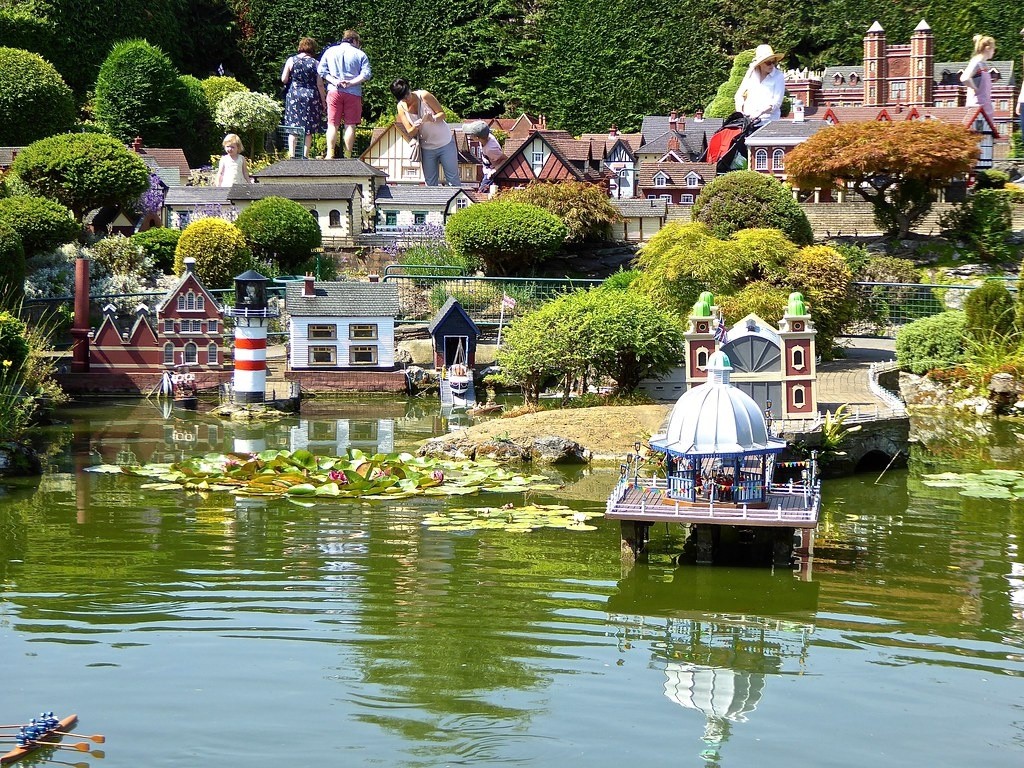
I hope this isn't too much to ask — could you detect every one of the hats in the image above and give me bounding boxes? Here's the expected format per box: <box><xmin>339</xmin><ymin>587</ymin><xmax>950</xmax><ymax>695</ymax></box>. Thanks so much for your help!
<box><xmin>462</xmin><ymin>121</ymin><xmax>489</xmax><ymax>137</ymax></box>
<box><xmin>752</xmin><ymin>45</ymin><xmax>785</xmax><ymax>67</ymax></box>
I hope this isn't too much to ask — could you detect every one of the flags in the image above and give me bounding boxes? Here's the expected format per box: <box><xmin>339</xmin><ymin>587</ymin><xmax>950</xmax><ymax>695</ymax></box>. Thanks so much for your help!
<box><xmin>712</xmin><ymin>317</ymin><xmax>727</xmax><ymax>344</ymax></box>
<box><xmin>504</xmin><ymin>293</ymin><xmax>516</xmax><ymax>309</ymax></box>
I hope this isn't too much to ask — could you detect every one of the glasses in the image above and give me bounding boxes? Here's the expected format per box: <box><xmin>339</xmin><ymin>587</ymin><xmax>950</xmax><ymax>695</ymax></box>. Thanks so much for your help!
<box><xmin>764</xmin><ymin>60</ymin><xmax>775</xmax><ymax>66</ymax></box>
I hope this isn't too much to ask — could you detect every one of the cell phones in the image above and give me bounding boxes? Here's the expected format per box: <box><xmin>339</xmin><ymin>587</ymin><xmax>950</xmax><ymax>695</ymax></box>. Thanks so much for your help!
<box><xmin>422</xmin><ymin>115</ymin><xmax>428</xmax><ymax>123</ymax></box>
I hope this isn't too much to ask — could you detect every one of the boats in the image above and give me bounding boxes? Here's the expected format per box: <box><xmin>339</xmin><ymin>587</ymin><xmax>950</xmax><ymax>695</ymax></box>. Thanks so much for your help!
<box><xmin>449</xmin><ymin>337</ymin><xmax>468</xmax><ymax>394</ymax></box>
<box><xmin>171</xmin><ymin>352</ymin><xmax>198</xmax><ymax>410</ymax></box>
<box><xmin>0</xmin><ymin>712</ymin><xmax>78</xmax><ymax>765</ymax></box>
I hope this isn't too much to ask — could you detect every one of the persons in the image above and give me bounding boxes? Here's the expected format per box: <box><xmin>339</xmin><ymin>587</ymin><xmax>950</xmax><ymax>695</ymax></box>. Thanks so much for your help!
<box><xmin>696</xmin><ymin>472</ymin><xmax>745</xmax><ymax>502</ymax></box>
<box><xmin>462</xmin><ymin>121</ymin><xmax>508</xmax><ymax>193</ymax></box>
<box><xmin>317</xmin><ymin>29</ymin><xmax>371</xmax><ymax>159</ymax></box>
<box><xmin>281</xmin><ymin>37</ymin><xmax>327</xmax><ymax>159</ymax></box>
<box><xmin>215</xmin><ymin>134</ymin><xmax>251</xmax><ymax>188</ymax></box>
<box><xmin>734</xmin><ymin>44</ymin><xmax>785</xmax><ymax>158</ymax></box>
<box><xmin>1016</xmin><ymin>28</ymin><xmax>1024</xmax><ymax>143</ymax></box>
<box><xmin>959</xmin><ymin>35</ymin><xmax>996</xmax><ymax>131</ymax></box>
<box><xmin>390</xmin><ymin>79</ymin><xmax>461</xmax><ymax>186</ymax></box>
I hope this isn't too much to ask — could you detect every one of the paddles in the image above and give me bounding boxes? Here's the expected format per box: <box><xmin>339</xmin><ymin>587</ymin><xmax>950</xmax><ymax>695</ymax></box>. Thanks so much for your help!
<box><xmin>24</xmin><ymin>746</ymin><xmax>106</xmax><ymax>768</ymax></box>
<box><xmin>0</xmin><ymin>723</ymin><xmax>106</xmax><ymax>753</ymax></box>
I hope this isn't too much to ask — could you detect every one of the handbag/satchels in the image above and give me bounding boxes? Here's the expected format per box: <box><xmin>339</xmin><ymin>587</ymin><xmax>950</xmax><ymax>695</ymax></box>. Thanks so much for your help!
<box><xmin>410</xmin><ymin>142</ymin><xmax>422</xmax><ymax>162</ymax></box>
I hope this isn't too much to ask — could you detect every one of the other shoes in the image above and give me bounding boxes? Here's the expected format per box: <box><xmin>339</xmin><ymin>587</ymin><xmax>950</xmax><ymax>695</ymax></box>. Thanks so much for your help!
<box><xmin>324</xmin><ymin>154</ymin><xmax>336</xmax><ymax>160</ymax></box>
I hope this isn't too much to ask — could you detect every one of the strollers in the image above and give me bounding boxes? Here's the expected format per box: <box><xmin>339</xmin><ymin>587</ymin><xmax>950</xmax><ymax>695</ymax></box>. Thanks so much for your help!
<box><xmin>696</xmin><ymin>106</ymin><xmax>772</xmax><ymax>176</ymax></box>
